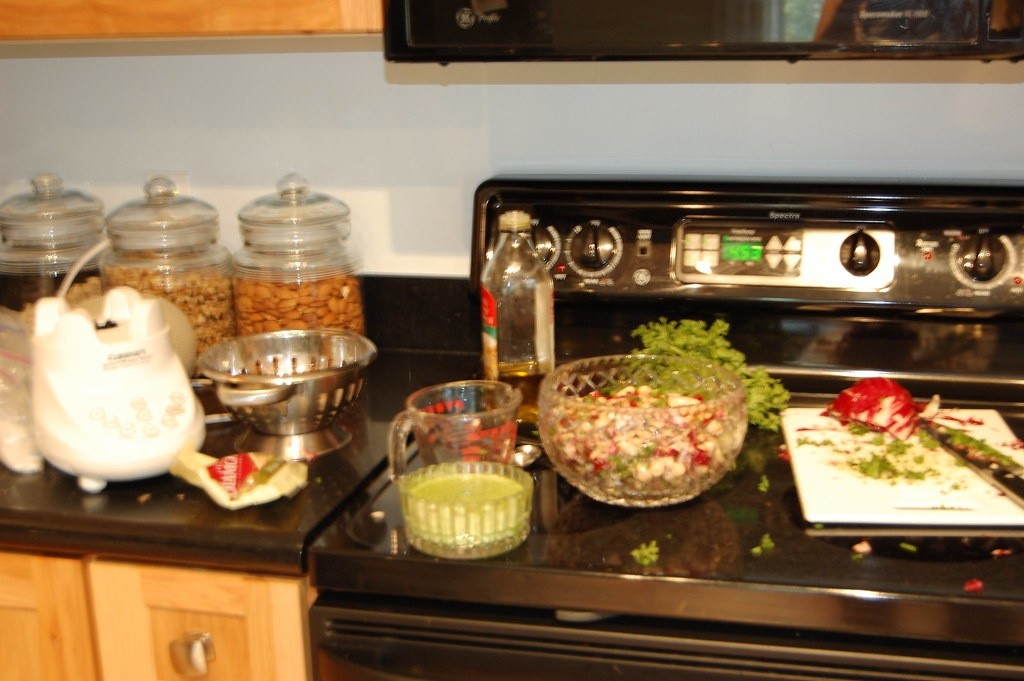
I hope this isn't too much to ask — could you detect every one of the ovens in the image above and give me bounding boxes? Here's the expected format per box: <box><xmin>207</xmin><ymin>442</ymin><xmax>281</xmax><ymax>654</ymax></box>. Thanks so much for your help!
<box><xmin>310</xmin><ymin>590</ymin><xmax>1024</xmax><ymax>681</ymax></box>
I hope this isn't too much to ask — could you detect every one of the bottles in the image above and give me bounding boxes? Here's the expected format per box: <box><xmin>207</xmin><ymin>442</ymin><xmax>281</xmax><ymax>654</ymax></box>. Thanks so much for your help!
<box><xmin>479</xmin><ymin>210</ymin><xmax>555</xmax><ymax>393</ymax></box>
<box><xmin>230</xmin><ymin>172</ymin><xmax>365</xmax><ymax>337</ymax></box>
<box><xmin>98</xmin><ymin>177</ymin><xmax>234</xmax><ymax>377</ymax></box>
<box><xmin>1</xmin><ymin>173</ymin><xmax>106</xmax><ymax>315</ymax></box>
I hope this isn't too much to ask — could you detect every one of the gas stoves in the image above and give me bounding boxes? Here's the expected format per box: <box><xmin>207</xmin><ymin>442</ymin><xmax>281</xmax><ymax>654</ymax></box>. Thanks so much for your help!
<box><xmin>311</xmin><ymin>176</ymin><xmax>1024</xmax><ymax>651</ymax></box>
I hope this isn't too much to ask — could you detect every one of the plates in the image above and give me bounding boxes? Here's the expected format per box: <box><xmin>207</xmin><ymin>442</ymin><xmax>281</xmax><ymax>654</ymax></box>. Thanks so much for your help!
<box><xmin>779</xmin><ymin>407</ymin><xmax>1024</xmax><ymax>527</ymax></box>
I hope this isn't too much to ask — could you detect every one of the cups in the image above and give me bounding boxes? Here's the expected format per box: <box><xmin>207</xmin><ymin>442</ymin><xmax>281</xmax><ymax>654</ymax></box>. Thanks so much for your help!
<box><xmin>386</xmin><ymin>380</ymin><xmax>524</xmax><ymax>484</ymax></box>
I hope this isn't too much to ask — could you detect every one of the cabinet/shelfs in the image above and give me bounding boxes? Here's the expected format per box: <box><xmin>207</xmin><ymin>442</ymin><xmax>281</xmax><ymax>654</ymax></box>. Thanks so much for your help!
<box><xmin>2</xmin><ymin>549</ymin><xmax>312</xmax><ymax>681</ymax></box>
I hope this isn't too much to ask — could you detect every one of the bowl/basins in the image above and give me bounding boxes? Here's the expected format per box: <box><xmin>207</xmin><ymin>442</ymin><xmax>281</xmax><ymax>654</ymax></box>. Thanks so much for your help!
<box><xmin>538</xmin><ymin>354</ymin><xmax>748</xmax><ymax>508</ymax></box>
<box><xmin>398</xmin><ymin>460</ymin><xmax>533</xmax><ymax>561</ymax></box>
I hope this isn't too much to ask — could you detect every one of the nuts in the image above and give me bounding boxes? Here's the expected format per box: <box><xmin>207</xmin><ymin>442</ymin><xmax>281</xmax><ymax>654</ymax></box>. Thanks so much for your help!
<box><xmin>64</xmin><ymin>259</ymin><xmax>365</xmax><ymax>376</ymax></box>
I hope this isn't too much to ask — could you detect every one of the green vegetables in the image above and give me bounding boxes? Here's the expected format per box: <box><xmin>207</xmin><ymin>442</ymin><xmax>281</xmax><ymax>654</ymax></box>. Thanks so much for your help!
<box><xmin>602</xmin><ymin>317</ymin><xmax>938</xmax><ymax>565</ymax></box>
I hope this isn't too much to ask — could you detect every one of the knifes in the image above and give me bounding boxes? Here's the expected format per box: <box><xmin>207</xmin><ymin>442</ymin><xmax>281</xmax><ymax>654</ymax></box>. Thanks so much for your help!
<box><xmin>921</xmin><ymin>419</ymin><xmax>1024</xmax><ymax>509</ymax></box>
